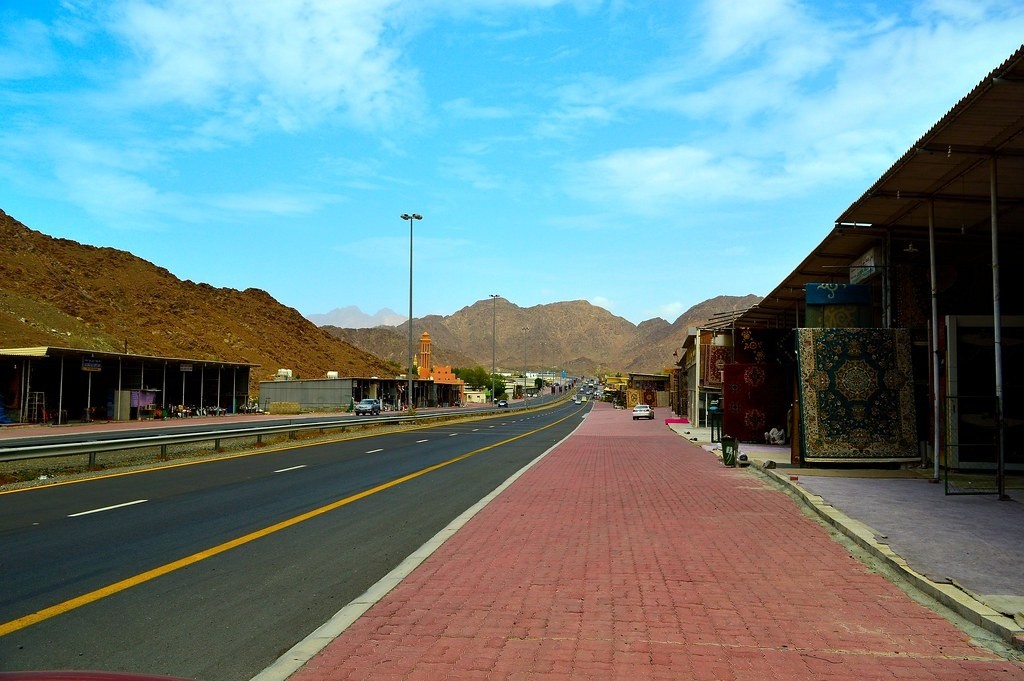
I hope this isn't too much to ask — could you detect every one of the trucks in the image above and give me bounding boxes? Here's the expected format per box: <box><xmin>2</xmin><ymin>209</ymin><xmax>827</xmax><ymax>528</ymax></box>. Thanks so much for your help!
<box><xmin>582</xmin><ymin>397</ymin><xmax>587</xmax><ymax>402</ymax></box>
<box><xmin>573</xmin><ymin>395</ymin><xmax>577</xmax><ymax>400</ymax></box>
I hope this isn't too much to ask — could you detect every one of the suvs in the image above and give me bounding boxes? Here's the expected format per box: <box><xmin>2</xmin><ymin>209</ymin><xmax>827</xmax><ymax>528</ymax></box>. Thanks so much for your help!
<box><xmin>354</xmin><ymin>399</ymin><xmax>380</xmax><ymax>416</ymax></box>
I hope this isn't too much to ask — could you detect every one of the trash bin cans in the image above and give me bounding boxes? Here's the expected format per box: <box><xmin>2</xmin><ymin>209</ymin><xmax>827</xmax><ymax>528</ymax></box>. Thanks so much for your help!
<box><xmin>721</xmin><ymin>437</ymin><xmax>739</xmax><ymax>468</ymax></box>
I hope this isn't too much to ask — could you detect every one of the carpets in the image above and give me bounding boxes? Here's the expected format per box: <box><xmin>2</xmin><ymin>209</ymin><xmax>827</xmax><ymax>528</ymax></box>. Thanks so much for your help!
<box><xmin>625</xmin><ymin>388</ymin><xmax>656</xmax><ymax>407</ymax></box>
<box><xmin>704</xmin><ymin>281</ymin><xmax>1024</xmax><ymax>493</ymax></box>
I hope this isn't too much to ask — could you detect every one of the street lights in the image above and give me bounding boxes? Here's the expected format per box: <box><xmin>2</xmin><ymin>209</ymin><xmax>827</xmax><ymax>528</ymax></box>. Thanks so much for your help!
<box><xmin>489</xmin><ymin>294</ymin><xmax>500</xmax><ymax>407</ymax></box>
<box><xmin>401</xmin><ymin>213</ymin><xmax>422</xmax><ymax>414</ymax></box>
<box><xmin>522</xmin><ymin>326</ymin><xmax>529</xmax><ymax>410</ymax></box>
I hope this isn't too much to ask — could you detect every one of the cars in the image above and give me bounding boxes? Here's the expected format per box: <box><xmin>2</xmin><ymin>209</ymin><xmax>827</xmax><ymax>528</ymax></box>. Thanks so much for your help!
<box><xmin>632</xmin><ymin>405</ymin><xmax>654</xmax><ymax>420</ymax></box>
<box><xmin>575</xmin><ymin>399</ymin><xmax>581</xmax><ymax>404</ymax></box>
<box><xmin>580</xmin><ymin>388</ymin><xmax>584</xmax><ymax>391</ymax></box>
<box><xmin>498</xmin><ymin>399</ymin><xmax>508</xmax><ymax>407</ymax></box>
